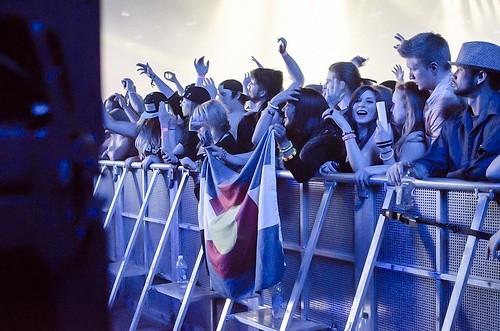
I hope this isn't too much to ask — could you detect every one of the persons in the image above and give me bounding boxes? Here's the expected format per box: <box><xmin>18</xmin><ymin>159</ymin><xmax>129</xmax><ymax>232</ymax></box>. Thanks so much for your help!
<box><xmin>101</xmin><ymin>32</ymin><xmax>500</xmax><ymax>261</ymax></box>
<box><xmin>321</xmin><ymin>85</ymin><xmax>393</xmax><ymax>174</ymax></box>
<box><xmin>179</xmin><ymin>99</ymin><xmax>237</xmax><ymax>164</ymax></box>
<box><xmin>251</xmin><ymin>85</ymin><xmax>331</xmax><ymax>183</ymax></box>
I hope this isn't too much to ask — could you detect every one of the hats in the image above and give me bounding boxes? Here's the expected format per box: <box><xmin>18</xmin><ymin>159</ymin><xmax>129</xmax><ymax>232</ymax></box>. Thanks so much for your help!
<box><xmin>447</xmin><ymin>41</ymin><xmax>500</xmax><ymax>70</ymax></box>
<box><xmin>144</xmin><ymin>92</ymin><xmax>167</xmax><ymax>113</ymax></box>
<box><xmin>184</xmin><ymin>87</ymin><xmax>211</xmax><ymax>106</ymax></box>
<box><xmin>220</xmin><ymin>80</ymin><xmax>249</xmax><ymax>101</ymax></box>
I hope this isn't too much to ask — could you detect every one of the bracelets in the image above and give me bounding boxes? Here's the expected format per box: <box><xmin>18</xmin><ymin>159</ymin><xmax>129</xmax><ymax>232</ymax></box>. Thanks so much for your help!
<box><xmin>219</xmin><ymin>149</ymin><xmax>227</xmax><ymax>159</ymax></box>
<box><xmin>277</xmin><ymin>141</ymin><xmax>293</xmax><ymax>153</ymax></box>
<box><xmin>281</xmin><ymin>52</ymin><xmax>288</xmax><ymax>57</ymax></box>
<box><xmin>375</xmin><ymin>139</ymin><xmax>393</xmax><ymax>147</ymax></box>
<box><xmin>160</xmin><ymin>128</ymin><xmax>168</xmax><ymax>131</ymax></box>
<box><xmin>150</xmin><ymin>75</ymin><xmax>155</xmax><ymax>87</ymax></box>
<box><xmin>341</xmin><ymin>130</ymin><xmax>354</xmax><ymax>135</ymax></box>
<box><xmin>267</xmin><ymin>102</ymin><xmax>278</xmax><ymax>110</ymax></box>
<box><xmin>282</xmin><ymin>148</ymin><xmax>297</xmax><ymax>162</ymax></box>
<box><xmin>198</xmin><ymin>76</ymin><xmax>204</xmax><ymax>80</ymax></box>
<box><xmin>380</xmin><ymin>149</ymin><xmax>393</xmax><ymax>161</ymax></box>
<box><xmin>342</xmin><ymin>134</ymin><xmax>356</xmax><ymax>140</ymax></box>
<box><xmin>266</xmin><ymin>109</ymin><xmax>275</xmax><ymax>116</ymax></box>
<box><xmin>169</xmin><ymin>128</ymin><xmax>176</xmax><ymax>131</ymax></box>
<box><xmin>128</xmin><ymin>89</ymin><xmax>135</xmax><ymax>94</ymax></box>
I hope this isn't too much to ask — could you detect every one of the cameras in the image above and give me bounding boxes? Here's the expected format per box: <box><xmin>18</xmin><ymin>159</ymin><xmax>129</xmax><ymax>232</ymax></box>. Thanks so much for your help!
<box><xmin>167</xmin><ymin>167</ymin><xmax>174</xmax><ymax>188</ymax></box>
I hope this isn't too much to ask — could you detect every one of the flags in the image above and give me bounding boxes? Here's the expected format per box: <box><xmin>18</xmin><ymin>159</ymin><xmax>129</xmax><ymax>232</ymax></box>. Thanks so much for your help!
<box><xmin>201</xmin><ymin>125</ymin><xmax>287</xmax><ymax>301</ymax></box>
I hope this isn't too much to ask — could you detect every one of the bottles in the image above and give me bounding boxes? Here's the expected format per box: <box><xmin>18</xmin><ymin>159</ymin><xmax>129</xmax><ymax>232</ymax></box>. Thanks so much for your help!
<box><xmin>175</xmin><ymin>255</ymin><xmax>188</xmax><ymax>288</ymax></box>
<box><xmin>396</xmin><ymin>161</ymin><xmax>416</xmax><ymax>210</ymax></box>
<box><xmin>357</xmin><ymin>313</ymin><xmax>371</xmax><ymax>331</ymax></box>
<box><xmin>271</xmin><ymin>282</ymin><xmax>285</xmax><ymax>321</ymax></box>
